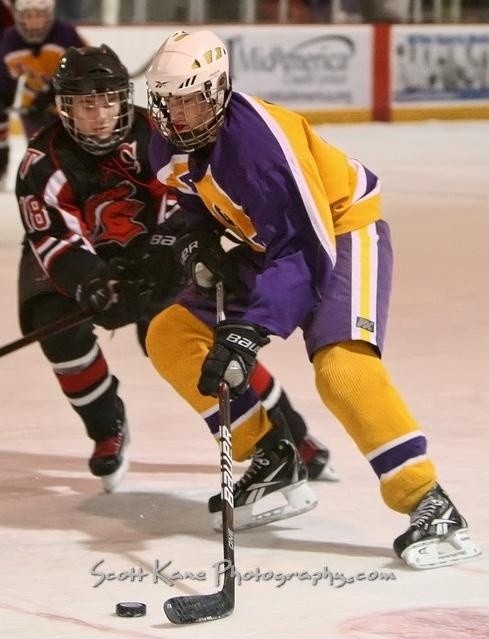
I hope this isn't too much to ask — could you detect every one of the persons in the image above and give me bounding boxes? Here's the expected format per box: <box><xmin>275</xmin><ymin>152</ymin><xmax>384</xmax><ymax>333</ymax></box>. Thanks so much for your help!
<box><xmin>1</xmin><ymin>0</ymin><xmax>87</xmax><ymax>175</ymax></box>
<box><xmin>16</xmin><ymin>42</ymin><xmax>330</xmax><ymax>480</ymax></box>
<box><xmin>146</xmin><ymin>29</ymin><xmax>468</xmax><ymax>558</ymax></box>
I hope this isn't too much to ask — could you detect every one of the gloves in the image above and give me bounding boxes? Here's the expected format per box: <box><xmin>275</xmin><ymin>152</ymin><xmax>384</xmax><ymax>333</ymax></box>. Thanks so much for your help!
<box><xmin>78</xmin><ymin>229</ymin><xmax>234</xmax><ymax>330</ymax></box>
<box><xmin>197</xmin><ymin>318</ymin><xmax>269</xmax><ymax>400</ymax></box>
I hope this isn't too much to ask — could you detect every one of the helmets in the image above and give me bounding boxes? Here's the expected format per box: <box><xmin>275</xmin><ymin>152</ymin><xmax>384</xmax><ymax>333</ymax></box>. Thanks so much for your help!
<box><xmin>12</xmin><ymin>1</ymin><xmax>56</xmax><ymax>45</ymax></box>
<box><xmin>145</xmin><ymin>29</ymin><xmax>234</xmax><ymax>154</ymax></box>
<box><xmin>49</xmin><ymin>45</ymin><xmax>136</xmax><ymax>157</ymax></box>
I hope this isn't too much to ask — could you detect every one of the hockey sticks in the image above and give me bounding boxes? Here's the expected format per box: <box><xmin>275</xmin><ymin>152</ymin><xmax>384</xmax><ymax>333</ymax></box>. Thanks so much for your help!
<box><xmin>164</xmin><ymin>282</ymin><xmax>235</xmax><ymax>625</ymax></box>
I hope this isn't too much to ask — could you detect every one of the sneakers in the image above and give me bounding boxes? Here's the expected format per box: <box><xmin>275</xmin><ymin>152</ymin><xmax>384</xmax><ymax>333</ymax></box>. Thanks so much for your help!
<box><xmin>206</xmin><ymin>410</ymin><xmax>330</xmax><ymax>516</ymax></box>
<box><xmin>87</xmin><ymin>400</ymin><xmax>129</xmax><ymax>479</ymax></box>
<box><xmin>392</xmin><ymin>481</ymin><xmax>469</xmax><ymax>559</ymax></box>
